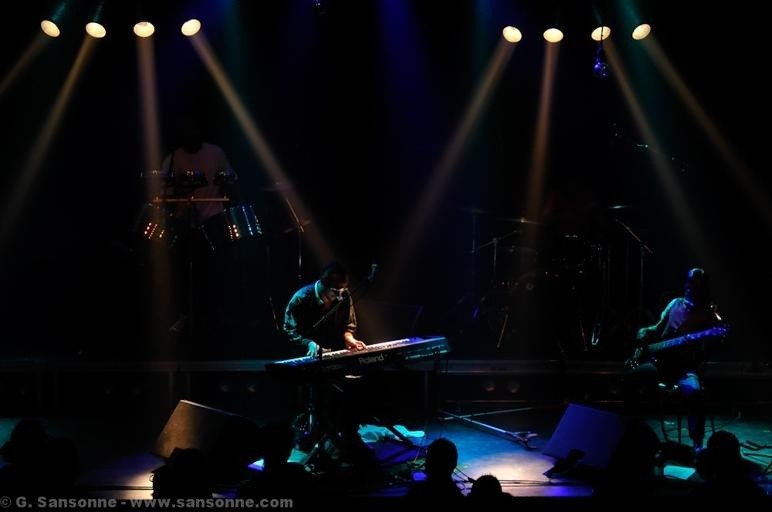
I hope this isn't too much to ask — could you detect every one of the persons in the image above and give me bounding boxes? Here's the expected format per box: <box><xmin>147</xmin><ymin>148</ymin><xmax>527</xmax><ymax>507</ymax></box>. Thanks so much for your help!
<box><xmin>632</xmin><ymin>267</ymin><xmax>729</xmax><ymax>466</ymax></box>
<box><xmin>0</xmin><ymin>414</ymin><xmax>772</xmax><ymax>512</ymax></box>
<box><xmin>282</xmin><ymin>261</ymin><xmax>367</xmax><ymax>445</ymax></box>
<box><xmin>539</xmin><ymin>164</ymin><xmax>603</xmax><ymax>236</ymax></box>
<box><xmin>149</xmin><ymin>123</ymin><xmax>239</xmax><ymax>338</ymax></box>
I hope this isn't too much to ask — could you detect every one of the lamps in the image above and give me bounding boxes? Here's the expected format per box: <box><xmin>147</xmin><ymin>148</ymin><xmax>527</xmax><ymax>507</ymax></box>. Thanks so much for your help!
<box><xmin>40</xmin><ymin>19</ymin><xmax>62</xmax><ymax>37</ymax></box>
<box><xmin>180</xmin><ymin>19</ymin><xmax>202</xmax><ymax>38</ymax></box>
<box><xmin>86</xmin><ymin>22</ymin><xmax>105</xmax><ymax>39</ymax></box>
<box><xmin>542</xmin><ymin>25</ymin><xmax>564</xmax><ymax>43</ymax></box>
<box><xmin>591</xmin><ymin>20</ymin><xmax>611</xmax><ymax>42</ymax></box>
<box><xmin>503</xmin><ymin>25</ymin><xmax>525</xmax><ymax>44</ymax></box>
<box><xmin>134</xmin><ymin>20</ymin><xmax>155</xmax><ymax>38</ymax></box>
<box><xmin>631</xmin><ymin>22</ymin><xmax>652</xmax><ymax>40</ymax></box>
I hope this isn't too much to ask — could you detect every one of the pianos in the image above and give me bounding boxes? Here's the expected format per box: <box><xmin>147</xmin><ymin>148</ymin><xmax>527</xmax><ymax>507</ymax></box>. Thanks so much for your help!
<box><xmin>265</xmin><ymin>336</ymin><xmax>450</xmax><ymax>387</ymax></box>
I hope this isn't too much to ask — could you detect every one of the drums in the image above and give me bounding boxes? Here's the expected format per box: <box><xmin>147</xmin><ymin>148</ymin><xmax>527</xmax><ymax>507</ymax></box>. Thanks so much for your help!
<box><xmin>200</xmin><ymin>204</ymin><xmax>262</xmax><ymax>250</ymax></box>
<box><xmin>142</xmin><ymin>219</ymin><xmax>180</xmax><ymax>250</ymax></box>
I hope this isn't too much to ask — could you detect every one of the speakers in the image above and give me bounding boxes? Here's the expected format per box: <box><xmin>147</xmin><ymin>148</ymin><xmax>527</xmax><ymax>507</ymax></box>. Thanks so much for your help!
<box><xmin>542</xmin><ymin>403</ymin><xmax>619</xmax><ymax>482</ymax></box>
<box><xmin>149</xmin><ymin>399</ymin><xmax>261</xmax><ymax>461</ymax></box>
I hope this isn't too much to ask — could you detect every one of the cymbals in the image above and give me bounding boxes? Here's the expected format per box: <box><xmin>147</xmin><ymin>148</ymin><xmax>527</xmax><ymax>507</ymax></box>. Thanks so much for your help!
<box><xmin>510</xmin><ymin>215</ymin><xmax>540</xmax><ymax>226</ymax></box>
<box><xmin>462</xmin><ymin>206</ymin><xmax>484</xmax><ymax>215</ymax></box>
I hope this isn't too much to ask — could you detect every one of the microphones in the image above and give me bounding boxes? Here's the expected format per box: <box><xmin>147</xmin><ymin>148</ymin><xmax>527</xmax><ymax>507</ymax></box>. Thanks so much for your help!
<box><xmin>371</xmin><ymin>263</ymin><xmax>379</xmax><ymax>278</ymax></box>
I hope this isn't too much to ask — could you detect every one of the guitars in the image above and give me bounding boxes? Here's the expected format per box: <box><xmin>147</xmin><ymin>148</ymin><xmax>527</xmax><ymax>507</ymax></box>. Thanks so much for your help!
<box><xmin>621</xmin><ymin>325</ymin><xmax>728</xmax><ymax>369</ymax></box>
